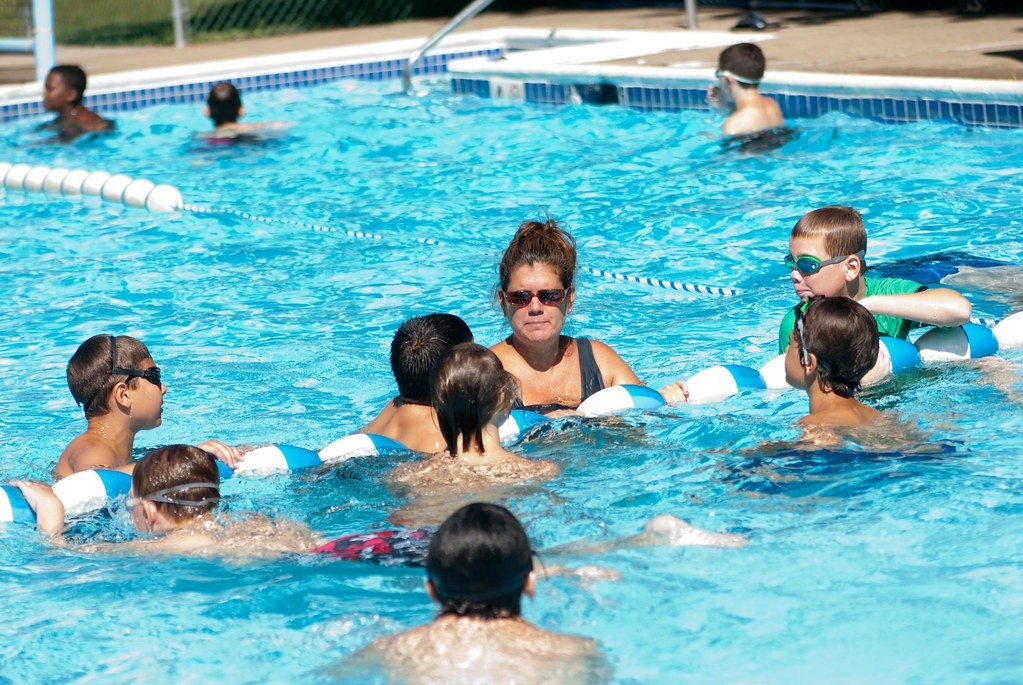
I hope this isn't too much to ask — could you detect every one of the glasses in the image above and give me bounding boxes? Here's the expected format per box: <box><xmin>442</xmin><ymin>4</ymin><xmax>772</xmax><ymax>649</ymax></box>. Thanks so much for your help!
<box><xmin>125</xmin><ymin>495</ymin><xmax>143</xmax><ymax>513</ymax></box>
<box><xmin>792</xmin><ymin>294</ymin><xmax>826</xmax><ymax>364</ymax></box>
<box><xmin>111</xmin><ymin>364</ymin><xmax>161</xmax><ymax>388</ymax></box>
<box><xmin>502</xmin><ymin>287</ymin><xmax>569</xmax><ymax>308</ymax></box>
<box><xmin>715</xmin><ymin>69</ymin><xmax>726</xmax><ymax>78</ymax></box>
<box><xmin>784</xmin><ymin>254</ymin><xmax>849</xmax><ymax>276</ymax></box>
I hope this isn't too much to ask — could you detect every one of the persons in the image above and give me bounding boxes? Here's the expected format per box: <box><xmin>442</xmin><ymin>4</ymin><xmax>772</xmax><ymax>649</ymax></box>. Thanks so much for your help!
<box><xmin>9</xmin><ymin>445</ymin><xmax>222</xmax><ymax>527</ymax></box>
<box><xmin>425</xmin><ymin>503</ymin><xmax>535</xmax><ymax>623</ymax></box>
<box><xmin>205</xmin><ymin>83</ymin><xmax>244</xmax><ymax>129</ymax></box>
<box><xmin>706</xmin><ymin>43</ymin><xmax>785</xmax><ymax>132</ymax></box>
<box><xmin>429</xmin><ymin>343</ymin><xmax>526</xmax><ymax>467</ymax></box>
<box><xmin>786</xmin><ymin>297</ymin><xmax>883</xmax><ymax>430</ymax></box>
<box><xmin>485</xmin><ymin>222</ymin><xmax>688</xmax><ymax>407</ymax></box>
<box><xmin>359</xmin><ymin>313</ymin><xmax>473</xmax><ymax>453</ymax></box>
<box><xmin>780</xmin><ymin>206</ymin><xmax>972</xmax><ymax>354</ymax></box>
<box><xmin>54</xmin><ymin>334</ymin><xmax>242</xmax><ymax>474</ymax></box>
<box><xmin>43</xmin><ymin>65</ymin><xmax>106</xmax><ymax>129</ymax></box>
